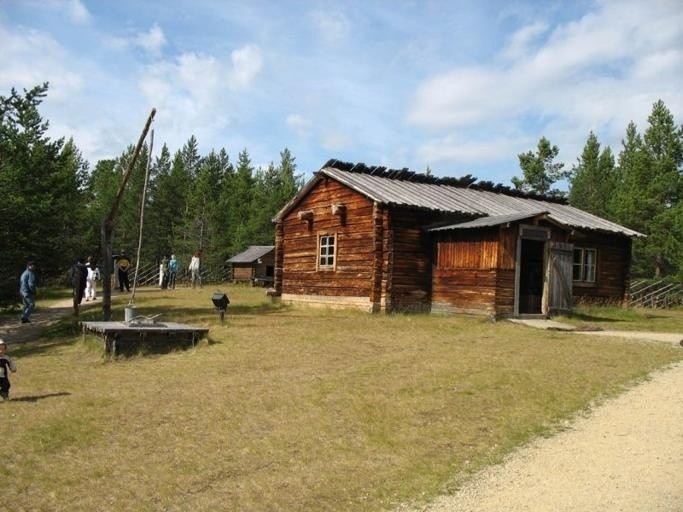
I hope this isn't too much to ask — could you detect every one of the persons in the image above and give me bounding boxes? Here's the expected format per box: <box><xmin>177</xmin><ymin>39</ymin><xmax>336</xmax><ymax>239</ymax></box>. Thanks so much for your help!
<box><xmin>116</xmin><ymin>249</ymin><xmax>131</xmax><ymax>291</ymax></box>
<box><xmin>188</xmin><ymin>249</ymin><xmax>203</xmax><ymax>287</ymax></box>
<box><xmin>19</xmin><ymin>261</ymin><xmax>38</xmax><ymax>324</ymax></box>
<box><xmin>68</xmin><ymin>258</ymin><xmax>88</xmax><ymax>316</ymax></box>
<box><xmin>0</xmin><ymin>339</ymin><xmax>16</xmax><ymax>401</ymax></box>
<box><xmin>82</xmin><ymin>255</ymin><xmax>101</xmax><ymax>302</ymax></box>
<box><xmin>168</xmin><ymin>254</ymin><xmax>178</xmax><ymax>290</ymax></box>
<box><xmin>160</xmin><ymin>256</ymin><xmax>169</xmax><ymax>289</ymax></box>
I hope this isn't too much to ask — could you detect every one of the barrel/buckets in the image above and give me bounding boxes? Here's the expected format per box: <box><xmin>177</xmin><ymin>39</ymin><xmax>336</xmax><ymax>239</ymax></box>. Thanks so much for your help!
<box><xmin>124</xmin><ymin>305</ymin><xmax>137</xmax><ymax>323</ymax></box>
